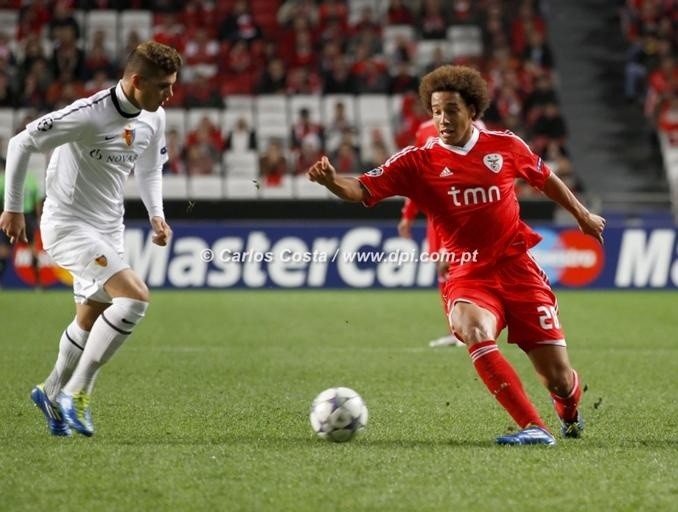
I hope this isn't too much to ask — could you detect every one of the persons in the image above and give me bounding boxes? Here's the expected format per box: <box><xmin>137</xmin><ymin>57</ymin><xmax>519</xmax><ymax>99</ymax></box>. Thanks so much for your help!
<box><xmin>307</xmin><ymin>64</ymin><xmax>606</xmax><ymax>446</ymax></box>
<box><xmin>397</xmin><ymin>118</ymin><xmax>486</xmax><ymax>349</ymax></box>
<box><xmin>618</xmin><ymin>0</ymin><xmax>678</xmax><ymax>147</ymax></box>
<box><xmin>0</xmin><ymin>0</ymin><xmax>575</xmax><ymax>190</ymax></box>
<box><xmin>0</xmin><ymin>40</ymin><xmax>184</xmax><ymax>437</ymax></box>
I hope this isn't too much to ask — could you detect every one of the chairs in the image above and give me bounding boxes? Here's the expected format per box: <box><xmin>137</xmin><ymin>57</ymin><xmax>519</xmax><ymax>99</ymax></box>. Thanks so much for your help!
<box><xmin>1</xmin><ymin>0</ymin><xmax>487</xmax><ymax>200</ymax></box>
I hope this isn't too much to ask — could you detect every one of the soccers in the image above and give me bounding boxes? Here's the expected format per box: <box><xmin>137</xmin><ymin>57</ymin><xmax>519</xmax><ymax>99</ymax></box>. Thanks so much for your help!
<box><xmin>307</xmin><ymin>385</ymin><xmax>368</xmax><ymax>444</ymax></box>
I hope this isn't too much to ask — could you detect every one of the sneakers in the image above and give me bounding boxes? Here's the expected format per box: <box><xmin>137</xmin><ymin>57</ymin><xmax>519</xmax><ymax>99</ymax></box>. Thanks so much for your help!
<box><xmin>496</xmin><ymin>422</ymin><xmax>556</xmax><ymax>447</ymax></box>
<box><xmin>549</xmin><ymin>395</ymin><xmax>583</xmax><ymax>439</ymax></box>
<box><xmin>31</xmin><ymin>384</ymin><xmax>95</xmax><ymax>437</ymax></box>
<box><xmin>430</xmin><ymin>335</ymin><xmax>464</xmax><ymax>346</ymax></box>
